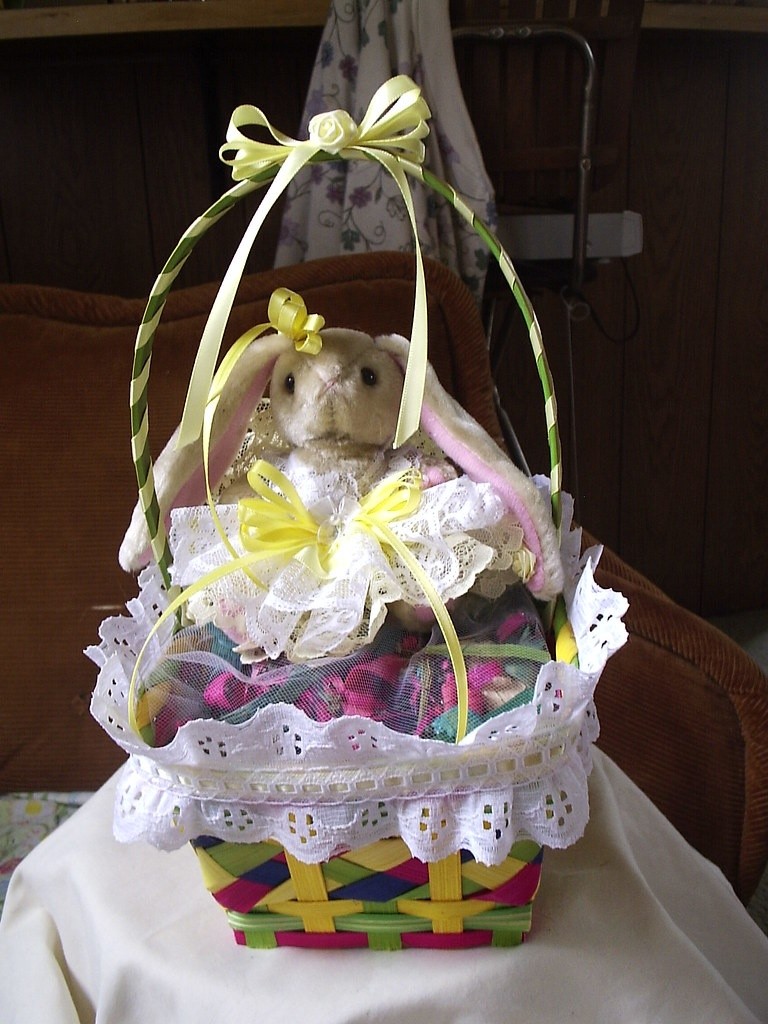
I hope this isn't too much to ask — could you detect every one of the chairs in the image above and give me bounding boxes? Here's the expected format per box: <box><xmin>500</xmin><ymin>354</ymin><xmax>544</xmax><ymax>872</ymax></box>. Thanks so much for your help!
<box><xmin>0</xmin><ymin>251</ymin><xmax>768</xmax><ymax>948</ymax></box>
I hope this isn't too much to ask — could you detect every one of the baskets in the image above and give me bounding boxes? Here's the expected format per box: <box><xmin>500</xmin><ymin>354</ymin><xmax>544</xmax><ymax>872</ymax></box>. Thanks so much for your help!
<box><xmin>83</xmin><ymin>76</ymin><xmax>629</xmax><ymax>948</ymax></box>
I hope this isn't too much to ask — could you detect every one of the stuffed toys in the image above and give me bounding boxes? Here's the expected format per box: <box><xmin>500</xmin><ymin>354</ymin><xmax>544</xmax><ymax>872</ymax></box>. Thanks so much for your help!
<box><xmin>116</xmin><ymin>329</ymin><xmax>566</xmax><ymax>644</ymax></box>
